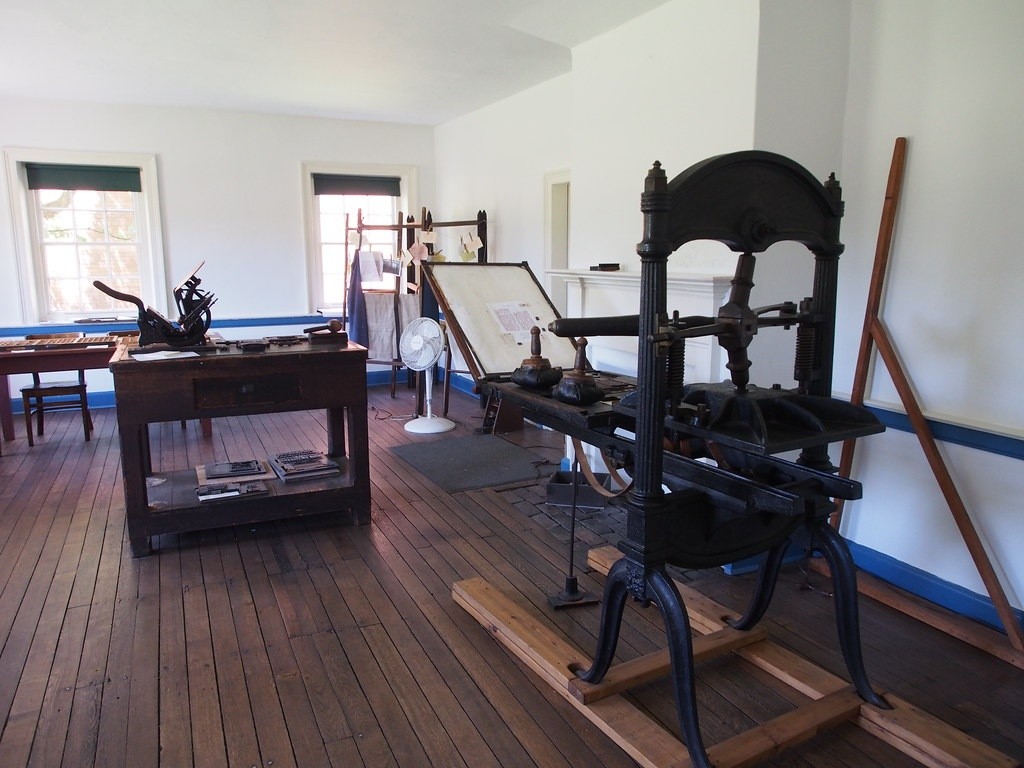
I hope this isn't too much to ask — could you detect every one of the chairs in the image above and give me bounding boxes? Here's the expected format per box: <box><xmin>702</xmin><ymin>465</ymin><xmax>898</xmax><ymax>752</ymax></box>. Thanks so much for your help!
<box><xmin>20</xmin><ymin>332</ymin><xmax>94</xmax><ymax>447</ymax></box>
<box><xmin>107</xmin><ymin>329</ymin><xmax>187</xmax><ymax>429</ymax></box>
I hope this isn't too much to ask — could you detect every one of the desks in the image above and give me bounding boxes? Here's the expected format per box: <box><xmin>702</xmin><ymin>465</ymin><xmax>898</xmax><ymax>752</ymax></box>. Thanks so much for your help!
<box><xmin>0</xmin><ymin>331</ymin><xmax>226</xmax><ymax>458</ymax></box>
<box><xmin>108</xmin><ymin>339</ymin><xmax>372</xmax><ymax>558</ymax></box>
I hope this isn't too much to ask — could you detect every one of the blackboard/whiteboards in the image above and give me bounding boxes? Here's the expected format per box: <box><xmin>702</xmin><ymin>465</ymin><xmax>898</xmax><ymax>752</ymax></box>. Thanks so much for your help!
<box><xmin>420</xmin><ymin>258</ymin><xmax>593</xmax><ymax>377</ymax></box>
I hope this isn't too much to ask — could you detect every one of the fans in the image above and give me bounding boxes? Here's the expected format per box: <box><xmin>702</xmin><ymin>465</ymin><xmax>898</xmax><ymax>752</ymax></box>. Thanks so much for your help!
<box><xmin>399</xmin><ymin>317</ymin><xmax>456</xmax><ymax>434</ymax></box>
